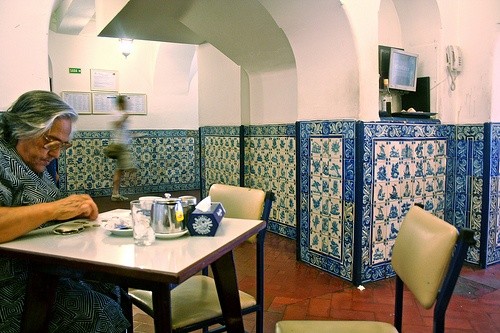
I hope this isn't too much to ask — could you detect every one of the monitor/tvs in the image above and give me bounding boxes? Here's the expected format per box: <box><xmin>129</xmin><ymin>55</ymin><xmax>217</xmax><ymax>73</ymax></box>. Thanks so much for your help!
<box><xmin>388</xmin><ymin>48</ymin><xmax>418</xmax><ymax>91</ymax></box>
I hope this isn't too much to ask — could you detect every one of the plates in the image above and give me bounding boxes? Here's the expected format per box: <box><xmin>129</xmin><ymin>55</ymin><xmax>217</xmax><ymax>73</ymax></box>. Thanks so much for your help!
<box><xmin>102</xmin><ymin>221</ymin><xmax>133</xmax><ymax>237</ymax></box>
<box><xmin>394</xmin><ymin>111</ymin><xmax>438</xmax><ymax>119</ymax></box>
<box><xmin>155</xmin><ymin>229</ymin><xmax>189</xmax><ymax>239</ymax></box>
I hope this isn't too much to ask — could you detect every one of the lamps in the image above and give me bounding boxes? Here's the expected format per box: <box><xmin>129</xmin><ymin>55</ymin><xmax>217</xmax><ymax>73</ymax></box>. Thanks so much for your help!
<box><xmin>119</xmin><ymin>38</ymin><xmax>133</xmax><ymax>58</ymax></box>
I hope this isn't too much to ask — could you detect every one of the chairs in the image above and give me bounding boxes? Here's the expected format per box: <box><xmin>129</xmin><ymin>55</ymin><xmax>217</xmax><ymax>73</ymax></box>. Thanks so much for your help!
<box><xmin>127</xmin><ymin>183</ymin><xmax>275</xmax><ymax>333</ymax></box>
<box><xmin>276</xmin><ymin>203</ymin><xmax>475</xmax><ymax>333</ymax></box>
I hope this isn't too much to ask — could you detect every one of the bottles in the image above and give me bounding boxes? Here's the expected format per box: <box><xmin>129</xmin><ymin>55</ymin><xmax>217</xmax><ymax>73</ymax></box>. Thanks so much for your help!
<box><xmin>381</xmin><ymin>78</ymin><xmax>392</xmax><ymax>116</ymax></box>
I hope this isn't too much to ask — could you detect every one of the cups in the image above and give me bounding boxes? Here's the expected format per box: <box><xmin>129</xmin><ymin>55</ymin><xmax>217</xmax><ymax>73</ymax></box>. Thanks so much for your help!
<box><xmin>179</xmin><ymin>195</ymin><xmax>197</xmax><ymax>223</ymax></box>
<box><xmin>139</xmin><ymin>196</ymin><xmax>163</xmax><ymax>220</ymax></box>
<box><xmin>130</xmin><ymin>199</ymin><xmax>156</xmax><ymax>246</ymax></box>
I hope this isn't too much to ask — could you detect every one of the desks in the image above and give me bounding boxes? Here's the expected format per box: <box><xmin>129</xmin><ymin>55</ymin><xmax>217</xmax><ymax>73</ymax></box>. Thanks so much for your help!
<box><xmin>0</xmin><ymin>209</ymin><xmax>267</xmax><ymax>333</ymax></box>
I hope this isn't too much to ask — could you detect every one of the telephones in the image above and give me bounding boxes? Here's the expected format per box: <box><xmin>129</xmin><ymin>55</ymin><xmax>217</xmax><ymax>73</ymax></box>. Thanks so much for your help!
<box><xmin>447</xmin><ymin>44</ymin><xmax>465</xmax><ymax>73</ymax></box>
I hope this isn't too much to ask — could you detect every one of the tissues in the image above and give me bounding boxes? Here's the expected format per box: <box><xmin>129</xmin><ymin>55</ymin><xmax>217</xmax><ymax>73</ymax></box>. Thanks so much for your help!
<box><xmin>186</xmin><ymin>195</ymin><xmax>226</xmax><ymax>237</ymax></box>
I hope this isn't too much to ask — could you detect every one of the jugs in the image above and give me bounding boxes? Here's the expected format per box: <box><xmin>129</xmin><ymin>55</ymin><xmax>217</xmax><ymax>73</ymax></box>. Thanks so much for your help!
<box><xmin>151</xmin><ymin>192</ymin><xmax>186</xmax><ymax>234</ymax></box>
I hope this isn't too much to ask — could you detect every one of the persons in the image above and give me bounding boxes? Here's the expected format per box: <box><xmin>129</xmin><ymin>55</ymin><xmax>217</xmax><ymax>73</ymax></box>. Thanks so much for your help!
<box><xmin>107</xmin><ymin>94</ymin><xmax>129</xmax><ymax>200</ymax></box>
<box><xmin>0</xmin><ymin>90</ymin><xmax>131</xmax><ymax>333</ymax></box>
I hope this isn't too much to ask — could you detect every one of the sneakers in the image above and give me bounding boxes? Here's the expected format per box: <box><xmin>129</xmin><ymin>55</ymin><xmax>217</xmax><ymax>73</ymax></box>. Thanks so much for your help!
<box><xmin>111</xmin><ymin>195</ymin><xmax>128</xmax><ymax>201</ymax></box>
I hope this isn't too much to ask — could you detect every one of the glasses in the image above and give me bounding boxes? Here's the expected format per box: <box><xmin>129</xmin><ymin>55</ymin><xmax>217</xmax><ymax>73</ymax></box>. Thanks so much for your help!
<box><xmin>37</xmin><ymin>129</ymin><xmax>72</xmax><ymax>151</ymax></box>
